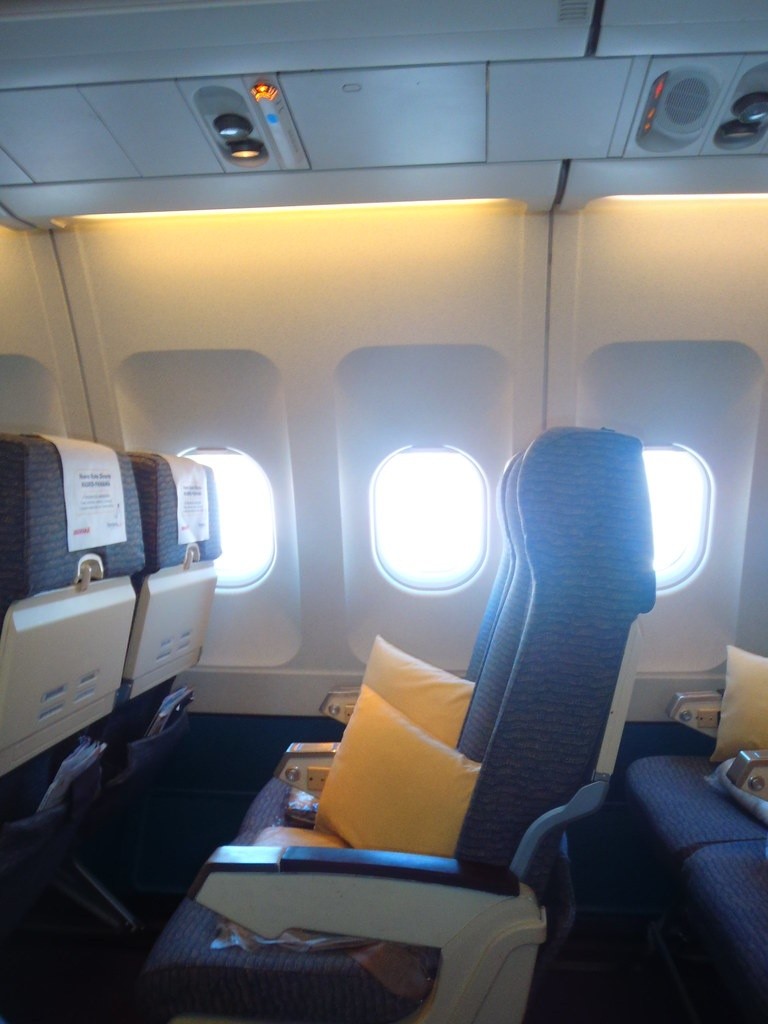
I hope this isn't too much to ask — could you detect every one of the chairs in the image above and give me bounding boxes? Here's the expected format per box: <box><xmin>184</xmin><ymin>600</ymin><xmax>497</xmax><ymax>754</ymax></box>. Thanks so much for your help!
<box><xmin>1</xmin><ymin>425</ymin><xmax>768</xmax><ymax>1024</ymax></box>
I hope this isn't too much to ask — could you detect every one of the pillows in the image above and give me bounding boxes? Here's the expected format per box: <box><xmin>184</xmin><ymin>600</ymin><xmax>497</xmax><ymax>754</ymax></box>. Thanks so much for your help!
<box><xmin>208</xmin><ymin>826</ymin><xmax>380</xmax><ymax>956</ymax></box>
<box><xmin>362</xmin><ymin>633</ymin><xmax>477</xmax><ymax>748</ymax></box>
<box><xmin>709</xmin><ymin>645</ymin><xmax>768</xmax><ymax>763</ymax></box>
<box><xmin>704</xmin><ymin>756</ymin><xmax>768</xmax><ymax>826</ymax></box>
<box><xmin>313</xmin><ymin>684</ymin><xmax>482</xmax><ymax>857</ymax></box>
